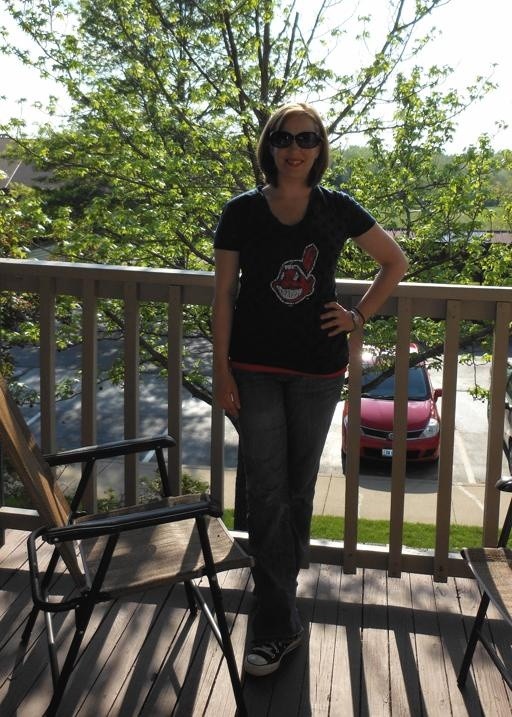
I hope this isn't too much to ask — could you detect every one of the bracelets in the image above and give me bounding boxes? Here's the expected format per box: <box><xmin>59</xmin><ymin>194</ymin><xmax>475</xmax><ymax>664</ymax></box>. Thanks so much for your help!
<box><xmin>348</xmin><ymin>304</ymin><xmax>364</xmax><ymax>331</ymax></box>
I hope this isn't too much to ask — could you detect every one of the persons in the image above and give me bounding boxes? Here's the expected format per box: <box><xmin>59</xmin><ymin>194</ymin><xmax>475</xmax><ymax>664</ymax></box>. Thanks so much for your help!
<box><xmin>211</xmin><ymin>103</ymin><xmax>409</xmax><ymax>675</ymax></box>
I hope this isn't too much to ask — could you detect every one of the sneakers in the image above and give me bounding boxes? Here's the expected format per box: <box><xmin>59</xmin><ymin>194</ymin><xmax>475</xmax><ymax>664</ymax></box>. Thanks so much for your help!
<box><xmin>241</xmin><ymin>625</ymin><xmax>308</xmax><ymax>678</ymax></box>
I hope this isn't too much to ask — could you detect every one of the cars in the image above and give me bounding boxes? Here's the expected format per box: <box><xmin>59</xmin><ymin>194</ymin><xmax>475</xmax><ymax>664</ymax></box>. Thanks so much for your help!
<box><xmin>342</xmin><ymin>341</ymin><xmax>443</xmax><ymax>463</ymax></box>
<box><xmin>487</xmin><ymin>357</ymin><xmax>512</xmax><ymax>477</ymax></box>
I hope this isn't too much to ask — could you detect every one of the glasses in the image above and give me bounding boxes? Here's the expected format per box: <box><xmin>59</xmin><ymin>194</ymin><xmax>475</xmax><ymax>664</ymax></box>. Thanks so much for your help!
<box><xmin>267</xmin><ymin>129</ymin><xmax>325</xmax><ymax>148</ymax></box>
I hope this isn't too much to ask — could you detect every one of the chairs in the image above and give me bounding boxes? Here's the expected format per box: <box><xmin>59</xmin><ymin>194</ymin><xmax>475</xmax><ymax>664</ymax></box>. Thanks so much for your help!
<box><xmin>0</xmin><ymin>378</ymin><xmax>254</xmax><ymax>717</ymax></box>
<box><xmin>458</xmin><ymin>476</ymin><xmax>512</xmax><ymax>696</ymax></box>
